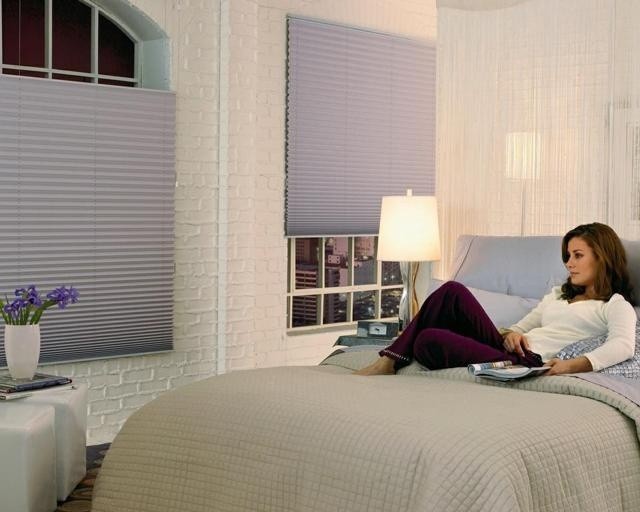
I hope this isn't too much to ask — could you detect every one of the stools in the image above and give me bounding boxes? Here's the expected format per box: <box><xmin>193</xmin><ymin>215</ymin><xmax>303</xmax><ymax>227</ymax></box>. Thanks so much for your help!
<box><xmin>0</xmin><ymin>401</ymin><xmax>59</xmax><ymax>512</ymax></box>
<box><xmin>14</xmin><ymin>382</ymin><xmax>88</xmax><ymax>501</ymax></box>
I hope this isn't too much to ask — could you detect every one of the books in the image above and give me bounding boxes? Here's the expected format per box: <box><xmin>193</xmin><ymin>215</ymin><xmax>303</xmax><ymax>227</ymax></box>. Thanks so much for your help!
<box><xmin>467</xmin><ymin>360</ymin><xmax>552</xmax><ymax>385</ymax></box>
<box><xmin>0</xmin><ymin>372</ymin><xmax>74</xmax><ymax>401</ymax></box>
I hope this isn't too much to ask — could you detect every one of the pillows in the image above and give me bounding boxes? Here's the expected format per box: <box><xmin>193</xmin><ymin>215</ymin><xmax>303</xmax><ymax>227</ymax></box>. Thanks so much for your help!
<box><xmin>427</xmin><ymin>276</ymin><xmax>538</xmax><ymax>326</ymax></box>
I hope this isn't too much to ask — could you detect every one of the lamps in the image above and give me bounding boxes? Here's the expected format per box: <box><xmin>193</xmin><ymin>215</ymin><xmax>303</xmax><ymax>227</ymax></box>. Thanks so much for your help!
<box><xmin>377</xmin><ymin>188</ymin><xmax>441</xmax><ymax>325</ymax></box>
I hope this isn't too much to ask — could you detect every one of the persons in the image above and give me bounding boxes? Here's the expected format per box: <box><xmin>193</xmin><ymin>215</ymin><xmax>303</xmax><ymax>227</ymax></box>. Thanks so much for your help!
<box><xmin>352</xmin><ymin>221</ymin><xmax>639</xmax><ymax>378</ymax></box>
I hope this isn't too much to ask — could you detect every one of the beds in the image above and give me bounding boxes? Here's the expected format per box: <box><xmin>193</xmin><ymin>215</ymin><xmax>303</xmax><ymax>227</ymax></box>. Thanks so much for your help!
<box><xmin>91</xmin><ymin>234</ymin><xmax>640</xmax><ymax>511</ymax></box>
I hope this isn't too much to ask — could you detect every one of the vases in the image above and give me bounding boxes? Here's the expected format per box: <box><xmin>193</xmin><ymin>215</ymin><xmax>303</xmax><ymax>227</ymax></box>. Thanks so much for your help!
<box><xmin>5</xmin><ymin>325</ymin><xmax>41</xmax><ymax>381</ymax></box>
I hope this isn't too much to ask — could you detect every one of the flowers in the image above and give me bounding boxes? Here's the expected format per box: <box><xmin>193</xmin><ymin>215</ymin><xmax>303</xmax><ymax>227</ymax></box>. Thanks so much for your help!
<box><xmin>0</xmin><ymin>283</ymin><xmax>79</xmax><ymax>326</ymax></box>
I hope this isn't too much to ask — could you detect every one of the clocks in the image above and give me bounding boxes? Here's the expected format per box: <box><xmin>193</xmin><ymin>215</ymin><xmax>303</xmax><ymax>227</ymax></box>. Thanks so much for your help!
<box><xmin>357</xmin><ymin>321</ymin><xmax>400</xmax><ymax>339</ymax></box>
